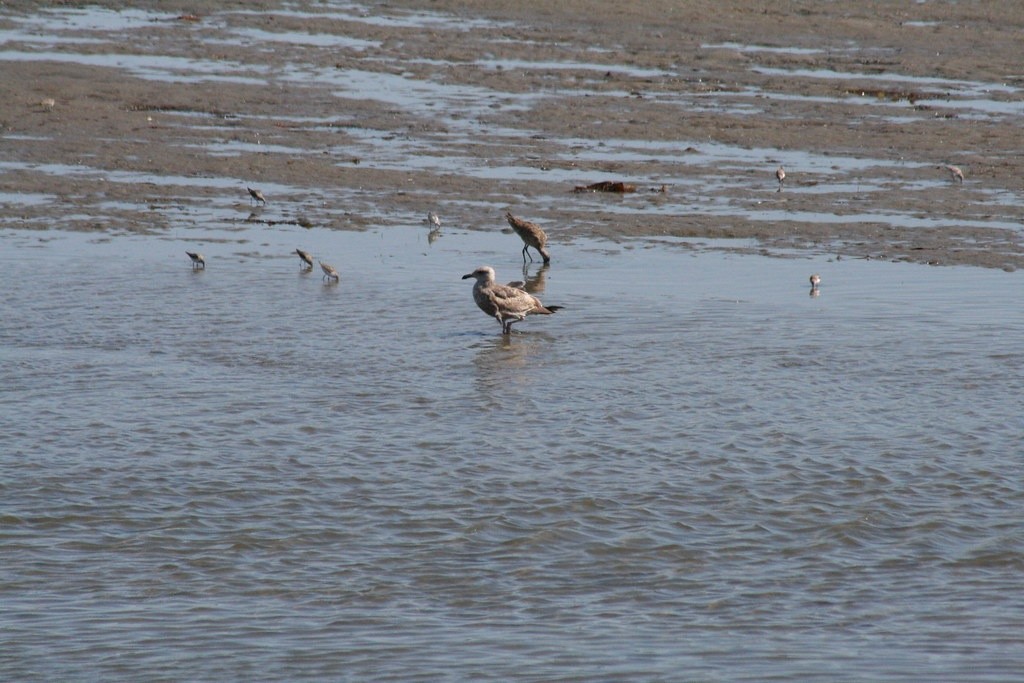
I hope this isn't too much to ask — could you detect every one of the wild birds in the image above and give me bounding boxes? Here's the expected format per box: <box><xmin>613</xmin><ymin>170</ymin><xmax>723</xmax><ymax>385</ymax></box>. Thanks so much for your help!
<box><xmin>428</xmin><ymin>210</ymin><xmax>442</xmax><ymax>233</ymax></box>
<box><xmin>504</xmin><ymin>212</ymin><xmax>551</xmax><ymax>265</ymax></box>
<box><xmin>246</xmin><ymin>185</ymin><xmax>267</xmax><ymax>207</ymax></box>
<box><xmin>776</xmin><ymin>165</ymin><xmax>786</xmax><ymax>193</ymax></box>
<box><xmin>460</xmin><ymin>264</ymin><xmax>566</xmax><ymax>336</ymax></box>
<box><xmin>808</xmin><ymin>272</ymin><xmax>822</xmax><ymax>289</ymax></box>
<box><xmin>185</xmin><ymin>250</ymin><xmax>207</xmax><ymax>271</ymax></box>
<box><xmin>318</xmin><ymin>259</ymin><xmax>340</xmax><ymax>285</ymax></box>
<box><xmin>942</xmin><ymin>163</ymin><xmax>966</xmax><ymax>182</ymax></box>
<box><xmin>294</xmin><ymin>248</ymin><xmax>314</xmax><ymax>270</ymax></box>
